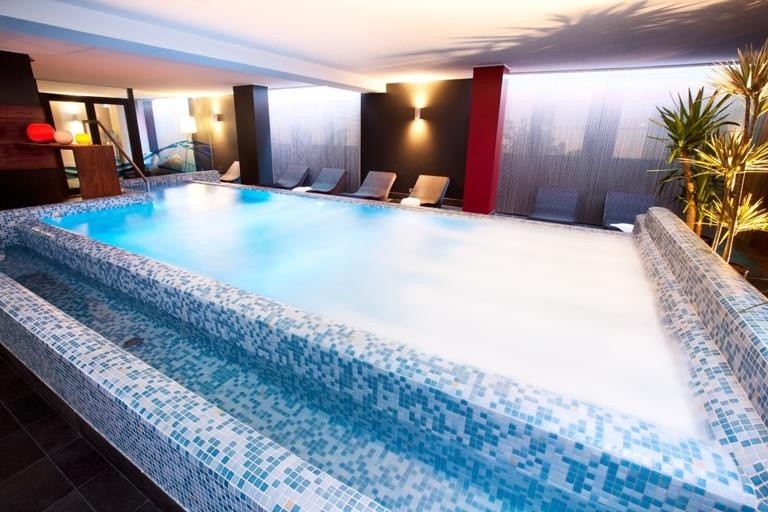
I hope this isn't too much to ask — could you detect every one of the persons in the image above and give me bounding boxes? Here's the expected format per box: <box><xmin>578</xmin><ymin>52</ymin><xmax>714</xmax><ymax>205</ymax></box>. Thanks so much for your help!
<box><xmin>143</xmin><ymin>150</ymin><xmax>159</xmax><ymax>168</ymax></box>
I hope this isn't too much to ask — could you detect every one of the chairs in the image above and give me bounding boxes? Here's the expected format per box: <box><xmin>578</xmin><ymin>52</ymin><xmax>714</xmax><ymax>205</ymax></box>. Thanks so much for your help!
<box><xmin>602</xmin><ymin>190</ymin><xmax>656</xmax><ymax>232</ymax></box>
<box><xmin>272</xmin><ymin>166</ymin><xmax>451</xmax><ymax>208</ymax></box>
<box><xmin>529</xmin><ymin>185</ymin><xmax>578</xmax><ymax>228</ymax></box>
<box><xmin>219</xmin><ymin>160</ymin><xmax>241</xmax><ymax>182</ymax></box>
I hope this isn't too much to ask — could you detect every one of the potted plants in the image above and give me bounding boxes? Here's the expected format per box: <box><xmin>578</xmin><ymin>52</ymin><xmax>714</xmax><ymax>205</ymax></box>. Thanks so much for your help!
<box><xmin>644</xmin><ymin>37</ymin><xmax>767</xmax><ymax>283</ymax></box>
